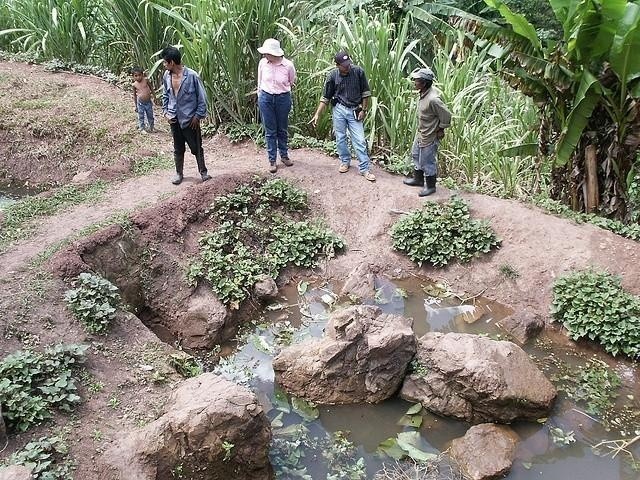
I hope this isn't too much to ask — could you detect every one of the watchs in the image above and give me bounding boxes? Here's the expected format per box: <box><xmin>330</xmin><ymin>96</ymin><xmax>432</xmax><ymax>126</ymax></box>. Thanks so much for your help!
<box><xmin>361</xmin><ymin>109</ymin><xmax>367</xmax><ymax>113</ymax></box>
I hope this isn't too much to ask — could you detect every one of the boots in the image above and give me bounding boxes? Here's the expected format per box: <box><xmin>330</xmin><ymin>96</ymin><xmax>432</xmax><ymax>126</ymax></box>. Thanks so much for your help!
<box><xmin>418</xmin><ymin>173</ymin><xmax>437</xmax><ymax>198</ymax></box>
<box><xmin>171</xmin><ymin>152</ymin><xmax>184</xmax><ymax>185</ymax></box>
<box><xmin>403</xmin><ymin>168</ymin><xmax>425</xmax><ymax>187</ymax></box>
<box><xmin>194</xmin><ymin>148</ymin><xmax>212</xmax><ymax>182</ymax></box>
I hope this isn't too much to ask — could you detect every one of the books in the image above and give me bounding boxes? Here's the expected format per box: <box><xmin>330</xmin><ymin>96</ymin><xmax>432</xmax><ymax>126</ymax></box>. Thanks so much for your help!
<box><xmin>352</xmin><ymin>108</ymin><xmax>362</xmax><ymax>120</ymax></box>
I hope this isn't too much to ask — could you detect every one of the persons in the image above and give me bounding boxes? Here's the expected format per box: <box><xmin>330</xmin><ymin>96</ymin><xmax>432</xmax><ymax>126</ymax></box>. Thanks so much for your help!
<box><xmin>160</xmin><ymin>47</ymin><xmax>212</xmax><ymax>184</ymax></box>
<box><xmin>308</xmin><ymin>53</ymin><xmax>376</xmax><ymax>182</ymax></box>
<box><xmin>132</xmin><ymin>66</ymin><xmax>158</xmax><ymax>131</ymax></box>
<box><xmin>402</xmin><ymin>68</ymin><xmax>450</xmax><ymax>196</ymax></box>
<box><xmin>257</xmin><ymin>38</ymin><xmax>297</xmax><ymax>173</ymax></box>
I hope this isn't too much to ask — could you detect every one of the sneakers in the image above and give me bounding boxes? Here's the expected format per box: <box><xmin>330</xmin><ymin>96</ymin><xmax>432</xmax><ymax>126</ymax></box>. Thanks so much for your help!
<box><xmin>338</xmin><ymin>161</ymin><xmax>351</xmax><ymax>174</ymax></box>
<box><xmin>359</xmin><ymin>168</ymin><xmax>377</xmax><ymax>182</ymax></box>
<box><xmin>269</xmin><ymin>161</ymin><xmax>277</xmax><ymax>172</ymax></box>
<box><xmin>280</xmin><ymin>156</ymin><xmax>294</xmax><ymax>167</ymax></box>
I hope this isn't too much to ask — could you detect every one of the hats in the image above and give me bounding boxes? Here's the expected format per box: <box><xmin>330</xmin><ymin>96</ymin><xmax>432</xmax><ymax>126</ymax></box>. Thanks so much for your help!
<box><xmin>256</xmin><ymin>38</ymin><xmax>284</xmax><ymax>57</ymax></box>
<box><xmin>409</xmin><ymin>68</ymin><xmax>435</xmax><ymax>82</ymax></box>
<box><xmin>334</xmin><ymin>51</ymin><xmax>353</xmax><ymax>67</ymax></box>
